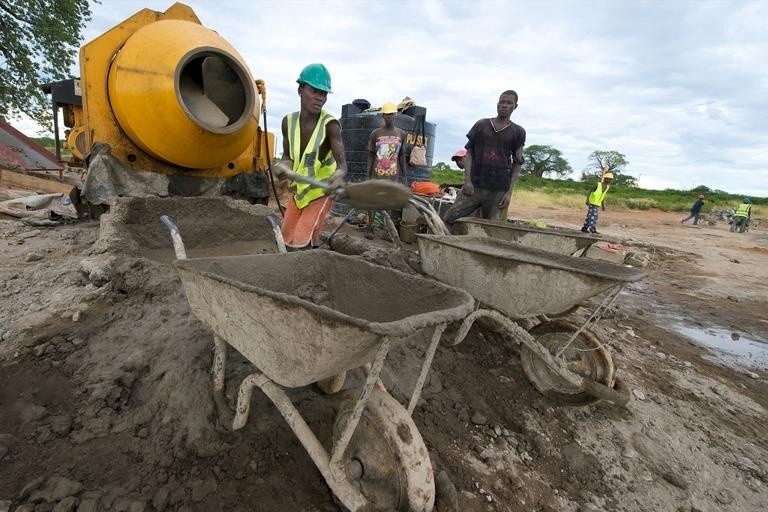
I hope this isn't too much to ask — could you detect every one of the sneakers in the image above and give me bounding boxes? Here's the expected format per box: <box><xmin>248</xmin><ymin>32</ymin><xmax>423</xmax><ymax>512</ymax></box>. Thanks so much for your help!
<box><xmin>383</xmin><ymin>232</ymin><xmax>391</xmax><ymax>242</ymax></box>
<box><xmin>582</xmin><ymin>227</ymin><xmax>600</xmax><ymax>234</ymax></box>
<box><xmin>364</xmin><ymin>227</ymin><xmax>375</xmax><ymax>239</ymax></box>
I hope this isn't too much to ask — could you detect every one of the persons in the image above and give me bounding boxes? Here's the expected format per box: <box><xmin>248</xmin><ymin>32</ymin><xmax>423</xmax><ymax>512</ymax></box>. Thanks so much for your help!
<box><xmin>682</xmin><ymin>195</ymin><xmax>705</xmax><ymax>225</ymax></box>
<box><xmin>452</xmin><ymin>151</ymin><xmax>469</xmax><ymax>169</ymax></box>
<box><xmin>272</xmin><ymin>63</ymin><xmax>350</xmax><ymax>251</ymax></box>
<box><xmin>730</xmin><ymin>197</ymin><xmax>751</xmax><ymax>232</ymax></box>
<box><xmin>364</xmin><ymin>101</ymin><xmax>410</xmax><ymax>240</ymax></box>
<box><xmin>443</xmin><ymin>89</ymin><xmax>527</xmax><ymax>222</ymax></box>
<box><xmin>582</xmin><ymin>172</ymin><xmax>616</xmax><ymax>234</ymax></box>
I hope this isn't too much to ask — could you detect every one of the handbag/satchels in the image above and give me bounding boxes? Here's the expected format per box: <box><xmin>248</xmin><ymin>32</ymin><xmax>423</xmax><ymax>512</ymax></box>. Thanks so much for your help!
<box><xmin>441</xmin><ymin>187</ymin><xmax>456</xmax><ymax>200</ymax></box>
<box><xmin>408</xmin><ymin>146</ymin><xmax>427</xmax><ymax>166</ymax></box>
<box><xmin>411</xmin><ymin>181</ymin><xmax>441</xmax><ymax>195</ymax></box>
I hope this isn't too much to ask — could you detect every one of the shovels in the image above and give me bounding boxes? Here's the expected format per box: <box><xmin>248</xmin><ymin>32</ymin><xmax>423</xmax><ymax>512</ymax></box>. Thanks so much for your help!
<box><xmin>282</xmin><ymin>168</ymin><xmax>411</xmax><ymax>210</ymax></box>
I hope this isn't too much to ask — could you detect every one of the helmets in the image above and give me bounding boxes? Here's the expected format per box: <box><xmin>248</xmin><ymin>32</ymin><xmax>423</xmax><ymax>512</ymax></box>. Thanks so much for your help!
<box><xmin>297</xmin><ymin>63</ymin><xmax>332</xmax><ymax>92</ymax></box>
<box><xmin>603</xmin><ymin>173</ymin><xmax>614</xmax><ymax>179</ymax></box>
<box><xmin>353</xmin><ymin>99</ymin><xmax>370</xmax><ymax>109</ymax></box>
<box><xmin>699</xmin><ymin>195</ymin><xmax>704</xmax><ymax>199</ymax></box>
<box><xmin>381</xmin><ymin>103</ymin><xmax>398</xmax><ymax>114</ymax></box>
<box><xmin>744</xmin><ymin>198</ymin><xmax>750</xmax><ymax>203</ymax></box>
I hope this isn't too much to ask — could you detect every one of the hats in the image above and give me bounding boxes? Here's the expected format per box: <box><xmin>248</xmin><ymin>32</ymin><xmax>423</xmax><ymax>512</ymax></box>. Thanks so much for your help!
<box><xmin>452</xmin><ymin>150</ymin><xmax>467</xmax><ymax>161</ymax></box>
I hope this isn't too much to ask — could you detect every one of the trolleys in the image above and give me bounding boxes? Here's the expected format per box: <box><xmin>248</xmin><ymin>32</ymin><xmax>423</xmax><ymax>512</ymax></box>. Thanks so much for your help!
<box><xmin>698</xmin><ymin>212</ymin><xmax>717</xmax><ymax>226</ymax></box>
<box><xmin>161</xmin><ymin>211</ymin><xmax>476</xmax><ymax>512</ymax></box>
<box><xmin>729</xmin><ymin>214</ymin><xmax>750</xmax><ymax>232</ymax></box>
